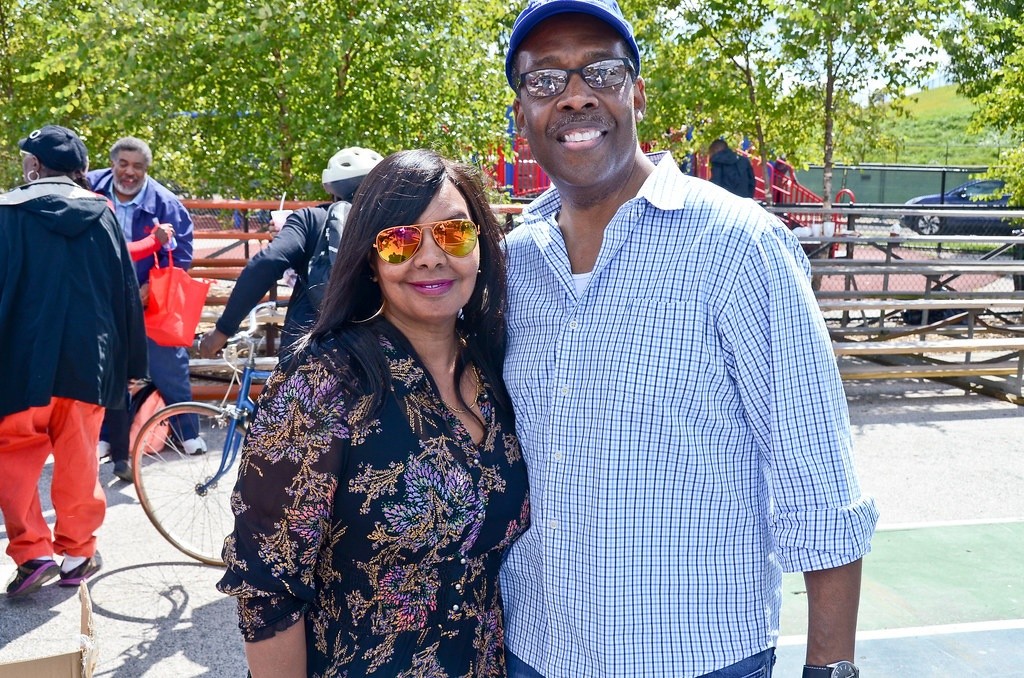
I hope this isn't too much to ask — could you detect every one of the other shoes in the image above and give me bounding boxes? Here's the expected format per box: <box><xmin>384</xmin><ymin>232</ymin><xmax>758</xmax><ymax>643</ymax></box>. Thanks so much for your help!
<box><xmin>114</xmin><ymin>462</ymin><xmax>133</xmax><ymax>483</ymax></box>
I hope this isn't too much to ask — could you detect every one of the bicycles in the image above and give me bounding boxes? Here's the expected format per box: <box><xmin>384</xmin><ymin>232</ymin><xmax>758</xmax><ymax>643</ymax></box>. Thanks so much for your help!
<box><xmin>132</xmin><ymin>300</ymin><xmax>291</xmax><ymax>568</ymax></box>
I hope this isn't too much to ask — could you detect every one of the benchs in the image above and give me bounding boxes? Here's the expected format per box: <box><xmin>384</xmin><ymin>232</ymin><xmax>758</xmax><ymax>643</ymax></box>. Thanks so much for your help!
<box><xmin>177</xmin><ymin>200</ymin><xmax>523</xmax><ymax>403</ymax></box>
<box><xmin>765</xmin><ymin>204</ymin><xmax>1024</xmax><ymax>399</ymax></box>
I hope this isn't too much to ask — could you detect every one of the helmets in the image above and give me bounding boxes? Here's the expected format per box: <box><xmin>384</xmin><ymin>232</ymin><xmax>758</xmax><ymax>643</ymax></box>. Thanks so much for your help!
<box><xmin>322</xmin><ymin>147</ymin><xmax>384</xmax><ymax>200</ymax></box>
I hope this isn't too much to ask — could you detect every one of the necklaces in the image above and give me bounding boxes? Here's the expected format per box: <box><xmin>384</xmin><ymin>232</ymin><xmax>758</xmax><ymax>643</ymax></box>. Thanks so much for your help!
<box><xmin>427</xmin><ymin>361</ymin><xmax>479</xmax><ymax>412</ymax></box>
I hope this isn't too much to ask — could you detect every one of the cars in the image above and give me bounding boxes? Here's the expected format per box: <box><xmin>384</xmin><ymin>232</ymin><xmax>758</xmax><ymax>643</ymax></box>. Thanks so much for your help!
<box><xmin>898</xmin><ymin>179</ymin><xmax>1016</xmax><ymax>235</ymax></box>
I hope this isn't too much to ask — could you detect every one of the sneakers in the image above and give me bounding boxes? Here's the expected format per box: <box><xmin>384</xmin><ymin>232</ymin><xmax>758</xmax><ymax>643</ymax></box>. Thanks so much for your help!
<box><xmin>6</xmin><ymin>558</ymin><xmax>61</xmax><ymax>598</ymax></box>
<box><xmin>97</xmin><ymin>440</ymin><xmax>112</xmax><ymax>466</ymax></box>
<box><xmin>57</xmin><ymin>550</ymin><xmax>102</xmax><ymax>586</ymax></box>
<box><xmin>174</xmin><ymin>436</ymin><xmax>207</xmax><ymax>454</ymax></box>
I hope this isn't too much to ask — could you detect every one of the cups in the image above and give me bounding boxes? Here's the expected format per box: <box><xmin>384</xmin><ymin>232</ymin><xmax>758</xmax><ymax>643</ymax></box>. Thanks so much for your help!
<box><xmin>271</xmin><ymin>210</ymin><xmax>293</xmax><ymax>231</ymax></box>
<box><xmin>812</xmin><ymin>222</ymin><xmax>835</xmax><ymax>237</ymax></box>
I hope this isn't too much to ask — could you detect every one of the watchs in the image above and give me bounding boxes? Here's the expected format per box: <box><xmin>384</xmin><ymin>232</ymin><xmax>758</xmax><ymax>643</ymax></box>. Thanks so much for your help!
<box><xmin>802</xmin><ymin>660</ymin><xmax>859</xmax><ymax>678</ymax></box>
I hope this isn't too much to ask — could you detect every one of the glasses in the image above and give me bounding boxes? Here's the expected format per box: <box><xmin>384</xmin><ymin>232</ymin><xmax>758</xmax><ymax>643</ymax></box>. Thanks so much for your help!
<box><xmin>19</xmin><ymin>151</ymin><xmax>32</xmax><ymax>157</ymax></box>
<box><xmin>373</xmin><ymin>219</ymin><xmax>481</xmax><ymax>264</ymax></box>
<box><xmin>517</xmin><ymin>57</ymin><xmax>635</xmax><ymax>99</ymax></box>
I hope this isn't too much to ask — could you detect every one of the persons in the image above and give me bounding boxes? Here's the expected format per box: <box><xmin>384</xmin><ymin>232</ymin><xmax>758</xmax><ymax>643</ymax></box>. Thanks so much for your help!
<box><xmin>0</xmin><ymin>126</ymin><xmax>176</xmax><ymax>599</ymax></box>
<box><xmin>216</xmin><ymin>150</ymin><xmax>530</xmax><ymax>678</ymax></box>
<box><xmin>457</xmin><ymin>0</ymin><xmax>877</xmax><ymax>678</ymax></box>
<box><xmin>706</xmin><ymin>139</ymin><xmax>755</xmax><ymax>199</ymax></box>
<box><xmin>201</xmin><ymin>146</ymin><xmax>384</xmax><ymax>362</ymax></box>
<box><xmin>87</xmin><ymin>137</ymin><xmax>206</xmax><ymax>461</ymax></box>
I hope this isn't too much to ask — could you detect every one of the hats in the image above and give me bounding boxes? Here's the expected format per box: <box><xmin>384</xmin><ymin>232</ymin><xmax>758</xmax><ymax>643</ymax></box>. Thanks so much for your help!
<box><xmin>20</xmin><ymin>125</ymin><xmax>89</xmax><ymax>173</ymax></box>
<box><xmin>506</xmin><ymin>0</ymin><xmax>641</xmax><ymax>94</ymax></box>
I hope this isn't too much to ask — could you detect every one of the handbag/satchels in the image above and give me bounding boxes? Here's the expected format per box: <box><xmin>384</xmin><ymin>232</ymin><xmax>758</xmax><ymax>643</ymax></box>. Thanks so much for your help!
<box><xmin>144</xmin><ymin>225</ymin><xmax>212</xmax><ymax>347</ymax></box>
